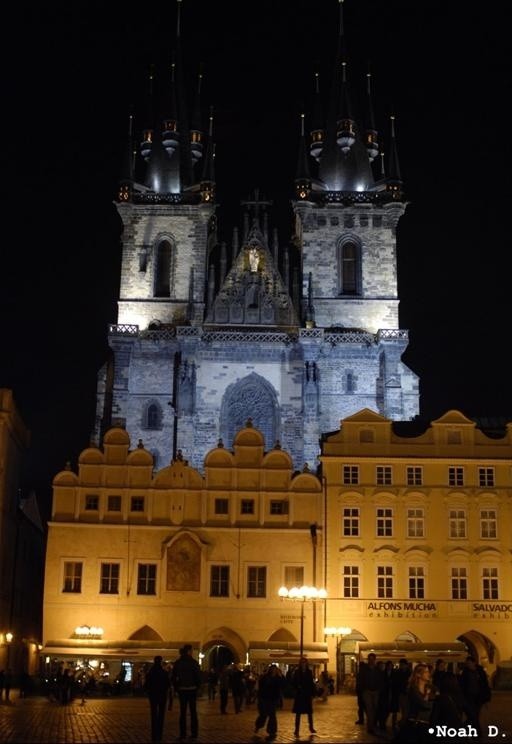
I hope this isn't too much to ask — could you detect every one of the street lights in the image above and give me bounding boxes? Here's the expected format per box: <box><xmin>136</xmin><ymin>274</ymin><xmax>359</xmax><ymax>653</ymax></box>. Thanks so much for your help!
<box><xmin>5</xmin><ymin>632</ymin><xmax>13</xmax><ymax>702</ymax></box>
<box><xmin>277</xmin><ymin>585</ymin><xmax>328</xmax><ymax>659</ymax></box>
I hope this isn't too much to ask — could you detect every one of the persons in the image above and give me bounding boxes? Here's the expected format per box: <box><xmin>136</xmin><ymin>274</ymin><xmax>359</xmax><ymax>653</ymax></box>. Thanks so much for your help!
<box><xmin>172</xmin><ymin>644</ymin><xmax>202</xmax><ymax>740</ymax></box>
<box><xmin>142</xmin><ymin>654</ymin><xmax>170</xmax><ymax>744</ymax></box>
<box><xmin>196</xmin><ymin>651</ymin><xmax>493</xmax><ymax>744</ymax></box>
